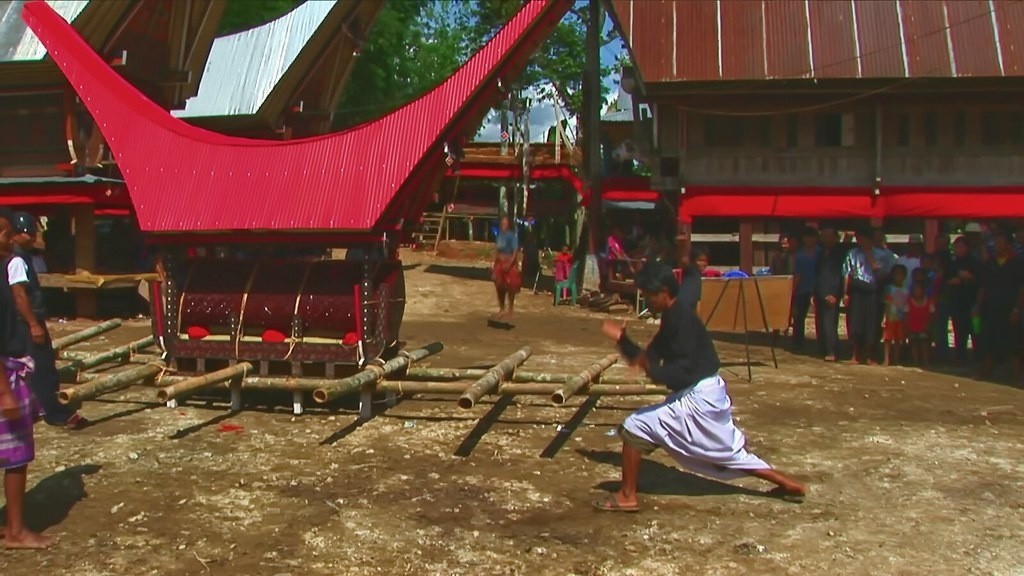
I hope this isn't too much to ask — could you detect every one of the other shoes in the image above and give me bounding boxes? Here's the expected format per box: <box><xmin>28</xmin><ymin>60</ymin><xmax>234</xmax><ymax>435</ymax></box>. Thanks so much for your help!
<box><xmin>848</xmin><ymin>355</ymin><xmax>860</xmax><ymax>365</ymax></box>
<box><xmin>823</xmin><ymin>353</ymin><xmax>839</xmax><ymax>361</ymax></box>
<box><xmin>64</xmin><ymin>414</ymin><xmax>87</xmax><ymax>429</ymax></box>
<box><xmin>490</xmin><ymin>314</ymin><xmax>512</xmax><ymax>323</ymax></box>
<box><xmin>867</xmin><ymin>358</ymin><xmax>879</xmax><ymax>367</ymax></box>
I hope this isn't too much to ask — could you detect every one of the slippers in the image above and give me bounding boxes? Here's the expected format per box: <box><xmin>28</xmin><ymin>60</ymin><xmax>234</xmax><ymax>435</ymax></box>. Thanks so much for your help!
<box><xmin>763</xmin><ymin>487</ymin><xmax>806</xmax><ymax>503</ymax></box>
<box><xmin>592</xmin><ymin>495</ymin><xmax>640</xmax><ymax>511</ymax></box>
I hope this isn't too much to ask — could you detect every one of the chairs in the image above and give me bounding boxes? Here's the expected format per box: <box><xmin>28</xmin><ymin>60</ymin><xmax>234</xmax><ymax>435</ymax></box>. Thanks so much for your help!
<box><xmin>554</xmin><ymin>259</ymin><xmax>581</xmax><ymax>306</ymax></box>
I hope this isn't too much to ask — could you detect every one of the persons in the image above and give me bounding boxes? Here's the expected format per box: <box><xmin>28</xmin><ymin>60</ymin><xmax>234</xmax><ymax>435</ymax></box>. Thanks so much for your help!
<box><xmin>492</xmin><ymin>209</ymin><xmax>1023</xmax><ymax>386</ymax></box>
<box><xmin>590</xmin><ymin>261</ymin><xmax>806</xmax><ymax>512</ymax></box>
<box><xmin>6</xmin><ymin>210</ymin><xmax>88</xmax><ymax>429</ymax></box>
<box><xmin>0</xmin><ymin>214</ymin><xmax>59</xmax><ymax>550</ymax></box>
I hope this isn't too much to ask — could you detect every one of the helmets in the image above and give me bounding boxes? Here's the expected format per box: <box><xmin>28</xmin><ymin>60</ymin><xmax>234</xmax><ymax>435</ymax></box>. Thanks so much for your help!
<box><xmin>965</xmin><ymin>222</ymin><xmax>982</xmax><ymax>233</ymax></box>
<box><xmin>12</xmin><ymin>212</ymin><xmax>41</xmax><ymax>235</ymax></box>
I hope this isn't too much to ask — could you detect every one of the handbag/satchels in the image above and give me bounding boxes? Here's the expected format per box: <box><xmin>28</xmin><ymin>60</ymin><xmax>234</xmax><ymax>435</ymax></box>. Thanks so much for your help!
<box><xmin>850</xmin><ymin>278</ymin><xmax>879</xmax><ymax>296</ymax></box>
